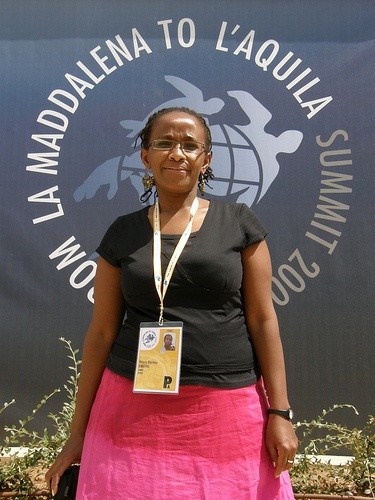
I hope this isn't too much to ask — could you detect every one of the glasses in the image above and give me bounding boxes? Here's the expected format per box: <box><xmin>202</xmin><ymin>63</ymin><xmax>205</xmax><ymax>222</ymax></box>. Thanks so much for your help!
<box><xmin>146</xmin><ymin>139</ymin><xmax>210</xmax><ymax>153</ymax></box>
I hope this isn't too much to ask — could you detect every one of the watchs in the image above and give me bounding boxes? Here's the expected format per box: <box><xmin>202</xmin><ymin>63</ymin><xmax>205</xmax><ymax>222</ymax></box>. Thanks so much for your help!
<box><xmin>266</xmin><ymin>408</ymin><xmax>294</xmax><ymax>422</ymax></box>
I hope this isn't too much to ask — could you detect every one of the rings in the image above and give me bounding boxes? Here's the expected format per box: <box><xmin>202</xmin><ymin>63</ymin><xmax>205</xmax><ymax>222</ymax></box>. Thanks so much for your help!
<box><xmin>287</xmin><ymin>459</ymin><xmax>295</xmax><ymax>463</ymax></box>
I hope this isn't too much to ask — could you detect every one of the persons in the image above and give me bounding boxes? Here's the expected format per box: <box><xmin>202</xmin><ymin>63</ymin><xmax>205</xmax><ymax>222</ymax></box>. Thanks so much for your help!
<box><xmin>162</xmin><ymin>334</ymin><xmax>175</xmax><ymax>351</ymax></box>
<box><xmin>43</xmin><ymin>107</ymin><xmax>297</xmax><ymax>500</ymax></box>
